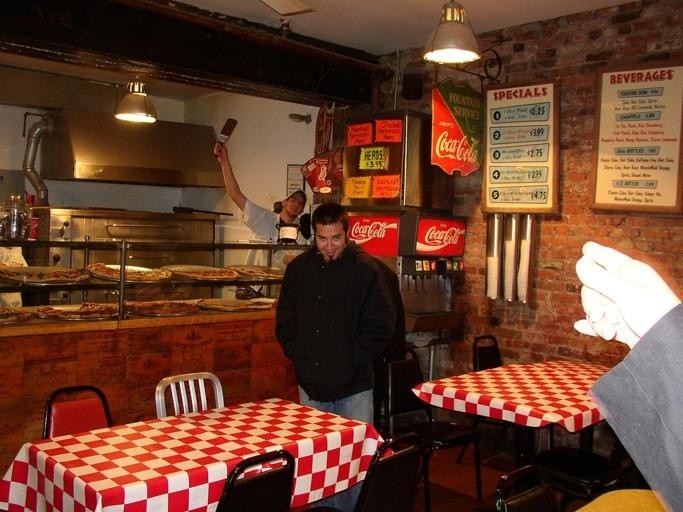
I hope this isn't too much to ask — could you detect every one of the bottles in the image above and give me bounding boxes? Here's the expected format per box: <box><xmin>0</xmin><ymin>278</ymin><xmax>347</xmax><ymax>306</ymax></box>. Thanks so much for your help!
<box><xmin>0</xmin><ymin>195</ymin><xmax>31</xmax><ymax>241</ymax></box>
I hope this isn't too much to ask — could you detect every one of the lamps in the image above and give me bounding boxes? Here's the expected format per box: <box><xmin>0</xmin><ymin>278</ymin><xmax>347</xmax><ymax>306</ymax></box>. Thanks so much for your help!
<box><xmin>110</xmin><ymin>60</ymin><xmax>159</xmax><ymax>126</ymax></box>
<box><xmin>420</xmin><ymin>0</ymin><xmax>484</xmax><ymax>69</ymax></box>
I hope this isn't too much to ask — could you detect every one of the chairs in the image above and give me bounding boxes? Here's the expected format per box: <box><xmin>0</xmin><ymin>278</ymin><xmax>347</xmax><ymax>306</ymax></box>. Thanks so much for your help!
<box><xmin>451</xmin><ymin>331</ymin><xmax>542</xmax><ymax>466</ymax></box>
<box><xmin>303</xmin><ymin>430</ymin><xmax>422</xmax><ymax>512</ymax></box>
<box><xmin>152</xmin><ymin>370</ymin><xmax>225</xmax><ymax>419</ymax></box>
<box><xmin>215</xmin><ymin>450</ymin><xmax>295</xmax><ymax>512</ymax></box>
<box><xmin>532</xmin><ymin>418</ymin><xmax>652</xmax><ymax>511</ymax></box>
<box><xmin>37</xmin><ymin>384</ymin><xmax>115</xmax><ymax>448</ymax></box>
<box><xmin>493</xmin><ymin>461</ymin><xmax>560</xmax><ymax>512</ymax></box>
<box><xmin>369</xmin><ymin>342</ymin><xmax>484</xmax><ymax>510</ymax></box>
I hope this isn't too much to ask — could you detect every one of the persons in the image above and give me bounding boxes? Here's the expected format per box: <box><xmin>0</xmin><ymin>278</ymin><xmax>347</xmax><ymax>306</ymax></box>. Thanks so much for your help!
<box><xmin>1</xmin><ymin>245</ymin><xmax>29</xmax><ymax>307</ymax></box>
<box><xmin>275</xmin><ymin>202</ymin><xmax>406</xmax><ymax>511</ymax></box>
<box><xmin>213</xmin><ymin>141</ymin><xmax>307</xmax><ymax>301</ymax></box>
<box><xmin>572</xmin><ymin>241</ymin><xmax>682</xmax><ymax>512</ymax></box>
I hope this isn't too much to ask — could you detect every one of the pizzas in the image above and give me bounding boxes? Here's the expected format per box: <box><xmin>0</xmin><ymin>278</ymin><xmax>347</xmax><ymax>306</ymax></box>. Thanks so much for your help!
<box><xmin>0</xmin><ymin>306</ymin><xmax>31</xmax><ymax>323</ymax></box>
<box><xmin>35</xmin><ymin>303</ymin><xmax>119</xmax><ymax>319</ymax></box>
<box><xmin>123</xmin><ymin>302</ymin><xmax>197</xmax><ymax>314</ymax></box>
<box><xmin>1</xmin><ymin>266</ymin><xmax>89</xmax><ymax>284</ymax></box>
<box><xmin>170</xmin><ymin>270</ymin><xmax>237</xmax><ymax>279</ymax></box>
<box><xmin>198</xmin><ymin>298</ymin><xmax>272</xmax><ymax>310</ymax></box>
<box><xmin>90</xmin><ymin>263</ymin><xmax>171</xmax><ymax>282</ymax></box>
<box><xmin>229</xmin><ymin>265</ymin><xmax>284</xmax><ymax>278</ymax></box>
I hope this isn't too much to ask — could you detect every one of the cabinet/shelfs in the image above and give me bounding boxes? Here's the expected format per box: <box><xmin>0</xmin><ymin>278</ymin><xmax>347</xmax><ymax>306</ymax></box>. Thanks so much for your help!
<box><xmin>1</xmin><ymin>233</ymin><xmax>315</xmax><ymax>339</ymax></box>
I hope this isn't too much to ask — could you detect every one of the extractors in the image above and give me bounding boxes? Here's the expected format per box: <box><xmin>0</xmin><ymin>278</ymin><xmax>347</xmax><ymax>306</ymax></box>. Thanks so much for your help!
<box><xmin>38</xmin><ymin>109</ymin><xmax>227</xmax><ymax>186</ymax></box>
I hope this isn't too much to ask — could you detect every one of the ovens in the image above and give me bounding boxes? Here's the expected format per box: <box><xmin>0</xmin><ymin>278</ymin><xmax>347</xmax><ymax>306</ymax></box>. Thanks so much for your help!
<box><xmin>24</xmin><ymin>207</ymin><xmax>223</xmax><ymax>303</ymax></box>
